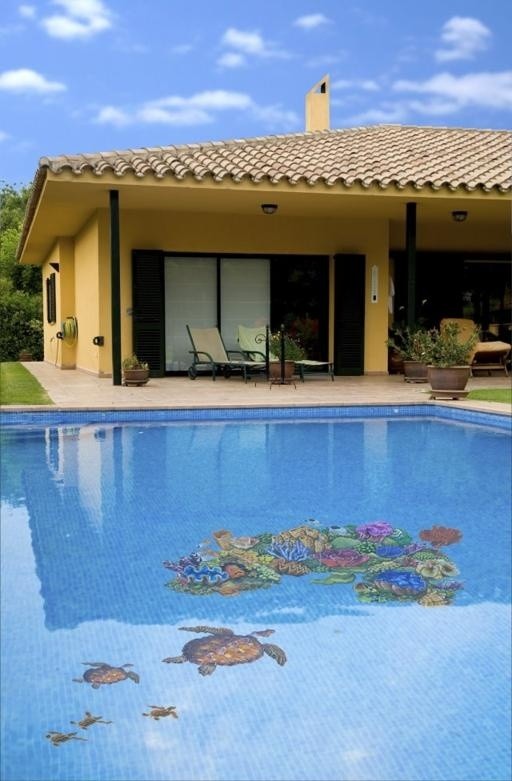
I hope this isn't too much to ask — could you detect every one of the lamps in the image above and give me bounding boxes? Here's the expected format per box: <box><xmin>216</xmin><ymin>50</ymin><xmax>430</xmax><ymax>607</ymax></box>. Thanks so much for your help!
<box><xmin>452</xmin><ymin>211</ymin><xmax>468</xmax><ymax>222</ymax></box>
<box><xmin>261</xmin><ymin>204</ymin><xmax>277</xmax><ymax>214</ymax></box>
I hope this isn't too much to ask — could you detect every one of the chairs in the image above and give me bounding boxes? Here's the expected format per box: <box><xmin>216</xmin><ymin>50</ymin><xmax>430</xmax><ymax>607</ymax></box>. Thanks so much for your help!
<box><xmin>440</xmin><ymin>318</ymin><xmax>512</xmax><ymax>377</ymax></box>
<box><xmin>186</xmin><ymin>324</ymin><xmax>334</xmax><ymax>384</ymax></box>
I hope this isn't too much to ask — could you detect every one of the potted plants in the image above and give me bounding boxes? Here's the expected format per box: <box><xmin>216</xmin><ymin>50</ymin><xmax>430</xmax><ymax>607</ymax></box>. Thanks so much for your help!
<box><xmin>123</xmin><ymin>354</ymin><xmax>149</xmax><ymax>387</ymax></box>
<box><xmin>385</xmin><ymin>321</ymin><xmax>484</xmax><ymax>400</ymax></box>
